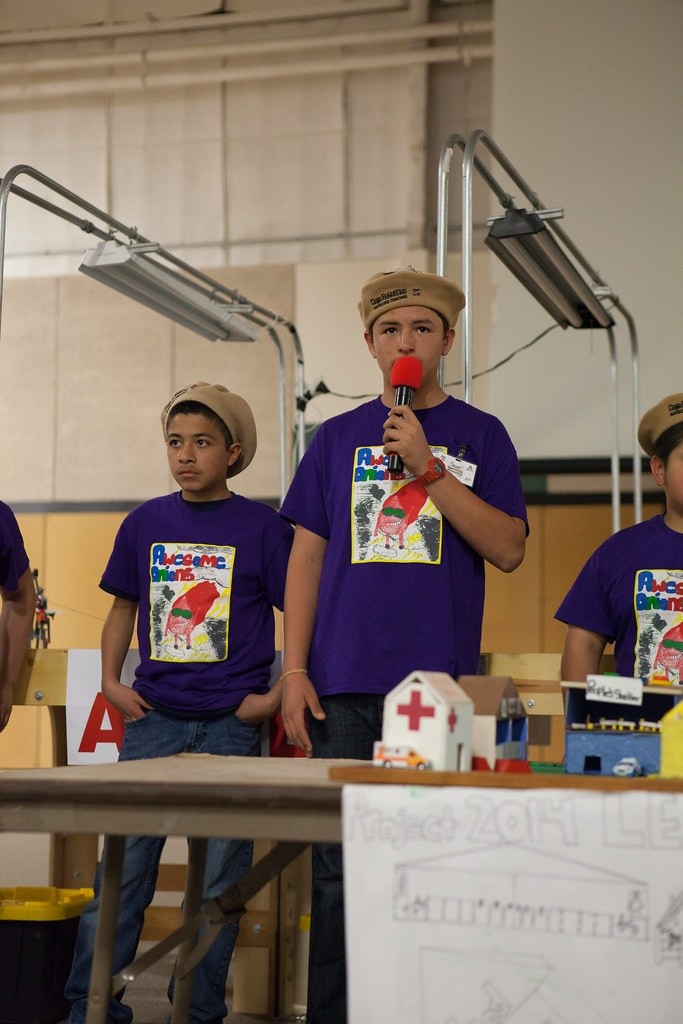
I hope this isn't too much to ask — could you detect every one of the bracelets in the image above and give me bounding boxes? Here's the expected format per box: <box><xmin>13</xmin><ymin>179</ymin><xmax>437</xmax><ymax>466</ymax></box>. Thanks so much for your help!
<box><xmin>279</xmin><ymin>669</ymin><xmax>306</xmax><ymax>682</ymax></box>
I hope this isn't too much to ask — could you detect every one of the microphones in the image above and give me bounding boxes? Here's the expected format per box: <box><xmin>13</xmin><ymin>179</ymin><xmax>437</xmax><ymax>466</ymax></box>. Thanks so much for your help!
<box><xmin>388</xmin><ymin>355</ymin><xmax>425</xmax><ymax>473</ymax></box>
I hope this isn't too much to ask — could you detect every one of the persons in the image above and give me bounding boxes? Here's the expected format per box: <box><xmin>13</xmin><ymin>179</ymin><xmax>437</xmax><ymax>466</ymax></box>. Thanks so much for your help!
<box><xmin>65</xmin><ymin>386</ymin><xmax>293</xmax><ymax>1024</ymax></box>
<box><xmin>553</xmin><ymin>392</ymin><xmax>683</xmax><ymax>688</ymax></box>
<box><xmin>0</xmin><ymin>503</ymin><xmax>36</xmax><ymax>732</ymax></box>
<box><xmin>280</xmin><ymin>269</ymin><xmax>529</xmax><ymax>1021</ymax></box>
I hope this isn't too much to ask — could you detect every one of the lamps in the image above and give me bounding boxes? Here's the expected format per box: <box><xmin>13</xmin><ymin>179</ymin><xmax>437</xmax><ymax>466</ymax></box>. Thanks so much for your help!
<box><xmin>484</xmin><ymin>207</ymin><xmax>617</xmax><ymax>330</ymax></box>
<box><xmin>78</xmin><ymin>240</ymin><xmax>261</xmax><ymax>344</ymax></box>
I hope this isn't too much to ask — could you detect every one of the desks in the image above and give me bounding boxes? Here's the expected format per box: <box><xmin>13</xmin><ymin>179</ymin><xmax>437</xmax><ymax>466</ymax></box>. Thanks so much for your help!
<box><xmin>0</xmin><ymin>752</ymin><xmax>683</xmax><ymax>1024</ymax></box>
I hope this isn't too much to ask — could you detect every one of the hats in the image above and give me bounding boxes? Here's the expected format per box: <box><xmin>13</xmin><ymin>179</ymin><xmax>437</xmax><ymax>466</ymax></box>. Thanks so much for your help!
<box><xmin>160</xmin><ymin>380</ymin><xmax>256</xmax><ymax>478</ymax></box>
<box><xmin>637</xmin><ymin>392</ymin><xmax>683</xmax><ymax>460</ymax></box>
<box><xmin>357</xmin><ymin>264</ymin><xmax>466</xmax><ymax>332</ymax></box>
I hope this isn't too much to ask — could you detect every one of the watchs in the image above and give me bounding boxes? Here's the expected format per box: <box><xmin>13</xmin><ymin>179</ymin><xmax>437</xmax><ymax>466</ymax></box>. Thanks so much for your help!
<box><xmin>419</xmin><ymin>458</ymin><xmax>446</xmax><ymax>486</ymax></box>
